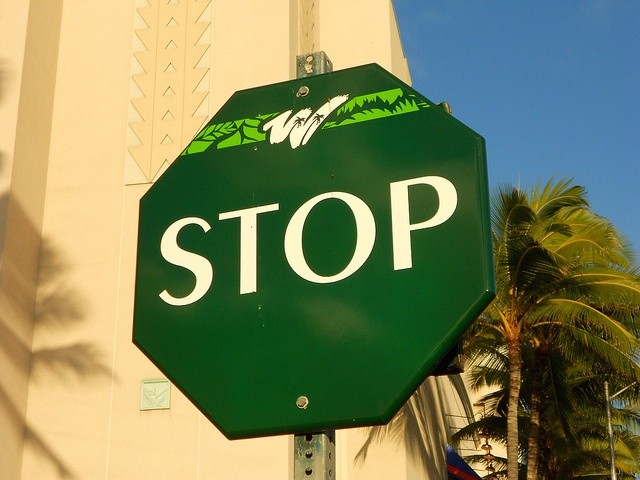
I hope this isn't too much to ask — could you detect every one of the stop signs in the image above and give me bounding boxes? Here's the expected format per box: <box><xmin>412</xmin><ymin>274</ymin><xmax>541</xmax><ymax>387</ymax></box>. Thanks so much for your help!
<box><xmin>131</xmin><ymin>63</ymin><xmax>497</xmax><ymax>441</ymax></box>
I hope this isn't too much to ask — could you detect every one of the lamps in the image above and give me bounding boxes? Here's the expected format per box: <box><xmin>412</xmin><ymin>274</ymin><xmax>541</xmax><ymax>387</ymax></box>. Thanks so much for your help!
<box><xmin>467</xmin><ymin>462</ymin><xmax>497</xmax><ymax>474</ymax></box>
<box><xmin>444</xmin><ymin>412</ymin><xmax>484</xmax><ymax>421</ymax></box>
<box><xmin>443</xmin><ymin>425</ymin><xmax>493</xmax><ymax>439</ymax></box>
<box><xmin>456</xmin><ymin>452</ymin><xmax>496</xmax><ymax>461</ymax></box>
<box><xmin>449</xmin><ymin>438</ymin><xmax>494</xmax><ymax>451</ymax></box>
